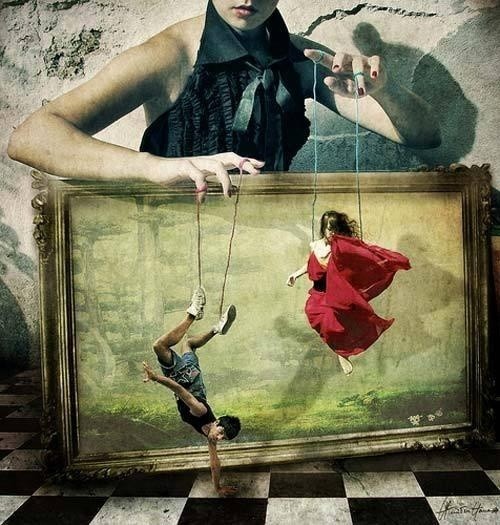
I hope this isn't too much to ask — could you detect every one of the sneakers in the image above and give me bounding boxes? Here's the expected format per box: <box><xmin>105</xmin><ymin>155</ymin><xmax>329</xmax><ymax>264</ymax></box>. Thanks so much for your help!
<box><xmin>216</xmin><ymin>304</ymin><xmax>236</xmax><ymax>335</ymax></box>
<box><xmin>186</xmin><ymin>287</ymin><xmax>206</xmax><ymax>320</ymax></box>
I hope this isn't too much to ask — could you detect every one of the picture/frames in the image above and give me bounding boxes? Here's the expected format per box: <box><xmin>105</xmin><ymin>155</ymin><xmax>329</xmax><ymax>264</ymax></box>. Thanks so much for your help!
<box><xmin>29</xmin><ymin>161</ymin><xmax>497</xmax><ymax>486</ymax></box>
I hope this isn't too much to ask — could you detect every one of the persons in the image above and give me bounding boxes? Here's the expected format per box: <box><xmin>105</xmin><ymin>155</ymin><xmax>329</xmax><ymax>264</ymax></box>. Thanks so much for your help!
<box><xmin>284</xmin><ymin>211</ymin><xmax>412</xmax><ymax>375</ymax></box>
<box><xmin>7</xmin><ymin>1</ymin><xmax>442</xmax><ymax>204</ymax></box>
<box><xmin>142</xmin><ymin>287</ymin><xmax>241</xmax><ymax>495</ymax></box>
